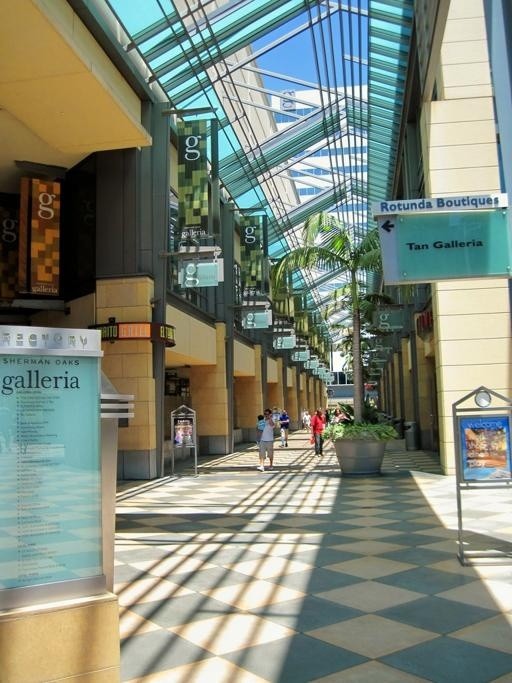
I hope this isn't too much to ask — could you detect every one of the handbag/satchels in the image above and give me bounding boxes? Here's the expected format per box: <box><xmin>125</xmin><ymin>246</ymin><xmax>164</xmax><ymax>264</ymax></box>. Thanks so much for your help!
<box><xmin>310</xmin><ymin>437</ymin><xmax>315</xmax><ymax>444</ymax></box>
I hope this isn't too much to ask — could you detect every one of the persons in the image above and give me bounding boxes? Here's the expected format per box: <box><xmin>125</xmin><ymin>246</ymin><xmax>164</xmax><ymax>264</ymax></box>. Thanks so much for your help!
<box><xmin>279</xmin><ymin>409</ymin><xmax>290</xmax><ymax>447</ymax></box>
<box><xmin>271</xmin><ymin>407</ymin><xmax>281</xmax><ymax>436</ymax></box>
<box><xmin>257</xmin><ymin>415</ymin><xmax>263</xmax><ymax>442</ymax></box>
<box><xmin>303</xmin><ymin>407</ymin><xmax>351</xmax><ymax>434</ymax></box>
<box><xmin>257</xmin><ymin>408</ymin><xmax>276</xmax><ymax>471</ymax></box>
<box><xmin>310</xmin><ymin>409</ymin><xmax>324</xmax><ymax>458</ymax></box>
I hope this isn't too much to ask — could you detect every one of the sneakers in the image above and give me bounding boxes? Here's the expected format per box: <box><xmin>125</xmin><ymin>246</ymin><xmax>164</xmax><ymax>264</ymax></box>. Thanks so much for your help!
<box><xmin>280</xmin><ymin>443</ymin><xmax>287</xmax><ymax>447</ymax></box>
<box><xmin>257</xmin><ymin>466</ymin><xmax>265</xmax><ymax>472</ymax></box>
<box><xmin>269</xmin><ymin>467</ymin><xmax>273</xmax><ymax>470</ymax></box>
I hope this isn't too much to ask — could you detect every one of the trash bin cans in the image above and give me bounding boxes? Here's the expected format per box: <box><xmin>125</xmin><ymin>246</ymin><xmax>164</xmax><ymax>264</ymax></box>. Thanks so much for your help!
<box><xmin>381</xmin><ymin>415</ymin><xmax>387</xmax><ymax>422</ymax></box>
<box><xmin>393</xmin><ymin>418</ymin><xmax>405</xmax><ymax>439</ymax></box>
<box><xmin>404</xmin><ymin>421</ymin><xmax>419</xmax><ymax>451</ymax></box>
<box><xmin>388</xmin><ymin>416</ymin><xmax>393</xmax><ymax>426</ymax></box>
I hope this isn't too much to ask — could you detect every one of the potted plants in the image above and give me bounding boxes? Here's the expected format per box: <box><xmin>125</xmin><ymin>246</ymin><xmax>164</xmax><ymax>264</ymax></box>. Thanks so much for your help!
<box><xmin>270</xmin><ymin>210</ymin><xmax>421</xmax><ymax>479</ymax></box>
